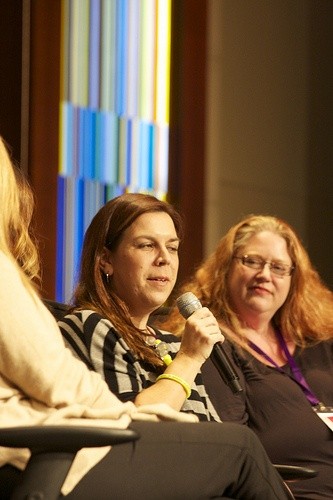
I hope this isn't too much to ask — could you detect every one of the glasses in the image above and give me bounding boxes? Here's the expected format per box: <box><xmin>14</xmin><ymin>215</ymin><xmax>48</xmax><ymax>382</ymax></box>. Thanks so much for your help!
<box><xmin>231</xmin><ymin>253</ymin><xmax>295</xmax><ymax>277</ymax></box>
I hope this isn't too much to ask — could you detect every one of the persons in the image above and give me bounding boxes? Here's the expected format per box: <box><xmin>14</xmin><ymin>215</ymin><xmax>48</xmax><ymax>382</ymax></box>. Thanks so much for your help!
<box><xmin>57</xmin><ymin>193</ymin><xmax>225</xmax><ymax>423</ymax></box>
<box><xmin>160</xmin><ymin>215</ymin><xmax>333</xmax><ymax>500</ymax></box>
<box><xmin>0</xmin><ymin>137</ymin><xmax>294</xmax><ymax>500</ymax></box>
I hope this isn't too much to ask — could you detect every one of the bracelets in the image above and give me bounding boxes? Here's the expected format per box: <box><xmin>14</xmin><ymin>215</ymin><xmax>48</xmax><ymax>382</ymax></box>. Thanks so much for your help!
<box><xmin>156</xmin><ymin>373</ymin><xmax>192</xmax><ymax>399</ymax></box>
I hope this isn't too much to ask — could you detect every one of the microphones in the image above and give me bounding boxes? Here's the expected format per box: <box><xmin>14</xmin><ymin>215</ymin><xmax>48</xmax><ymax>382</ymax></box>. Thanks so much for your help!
<box><xmin>177</xmin><ymin>291</ymin><xmax>244</xmax><ymax>396</ymax></box>
